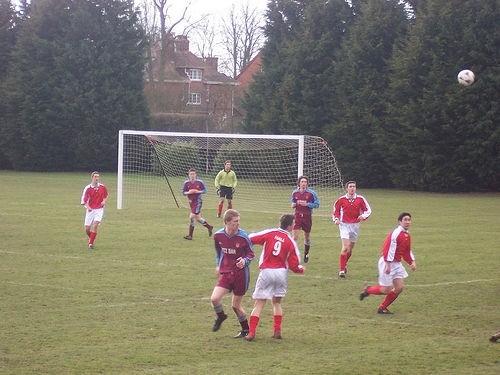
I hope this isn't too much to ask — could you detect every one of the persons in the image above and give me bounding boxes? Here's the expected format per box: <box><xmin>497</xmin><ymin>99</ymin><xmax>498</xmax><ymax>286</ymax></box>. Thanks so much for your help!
<box><xmin>333</xmin><ymin>181</ymin><xmax>371</xmax><ymax>278</ymax></box>
<box><xmin>244</xmin><ymin>214</ymin><xmax>305</xmax><ymax>341</ymax></box>
<box><xmin>81</xmin><ymin>171</ymin><xmax>109</xmax><ymax>248</ymax></box>
<box><xmin>489</xmin><ymin>328</ymin><xmax>500</xmax><ymax>342</ymax></box>
<box><xmin>182</xmin><ymin>168</ymin><xmax>213</xmax><ymax>240</ymax></box>
<box><xmin>290</xmin><ymin>176</ymin><xmax>320</xmax><ymax>263</ymax></box>
<box><xmin>214</xmin><ymin>160</ymin><xmax>237</xmax><ymax>218</ymax></box>
<box><xmin>360</xmin><ymin>212</ymin><xmax>416</xmax><ymax>314</ymax></box>
<box><xmin>211</xmin><ymin>209</ymin><xmax>256</xmax><ymax>338</ymax></box>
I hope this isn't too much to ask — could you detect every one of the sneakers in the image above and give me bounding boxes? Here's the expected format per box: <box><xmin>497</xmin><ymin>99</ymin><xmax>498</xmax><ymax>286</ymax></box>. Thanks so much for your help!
<box><xmin>272</xmin><ymin>331</ymin><xmax>281</xmax><ymax>339</ymax></box>
<box><xmin>244</xmin><ymin>333</ymin><xmax>256</xmax><ymax>342</ymax></box>
<box><xmin>208</xmin><ymin>226</ymin><xmax>213</xmax><ymax>237</ymax></box>
<box><xmin>232</xmin><ymin>326</ymin><xmax>249</xmax><ymax>339</ymax></box>
<box><xmin>338</xmin><ymin>267</ymin><xmax>348</xmax><ymax>277</ymax></box>
<box><xmin>88</xmin><ymin>244</ymin><xmax>93</xmax><ymax>249</ymax></box>
<box><xmin>377</xmin><ymin>306</ymin><xmax>394</xmax><ymax>314</ymax></box>
<box><xmin>216</xmin><ymin>211</ymin><xmax>221</xmax><ymax>218</ymax></box>
<box><xmin>184</xmin><ymin>235</ymin><xmax>192</xmax><ymax>240</ymax></box>
<box><xmin>304</xmin><ymin>250</ymin><xmax>309</xmax><ymax>263</ymax></box>
<box><xmin>212</xmin><ymin>313</ymin><xmax>227</xmax><ymax>332</ymax></box>
<box><xmin>359</xmin><ymin>285</ymin><xmax>369</xmax><ymax>301</ymax></box>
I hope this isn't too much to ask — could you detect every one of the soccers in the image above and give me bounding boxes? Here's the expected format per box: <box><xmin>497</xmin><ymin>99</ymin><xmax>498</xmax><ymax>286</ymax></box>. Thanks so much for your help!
<box><xmin>458</xmin><ymin>70</ymin><xmax>474</xmax><ymax>86</ymax></box>
